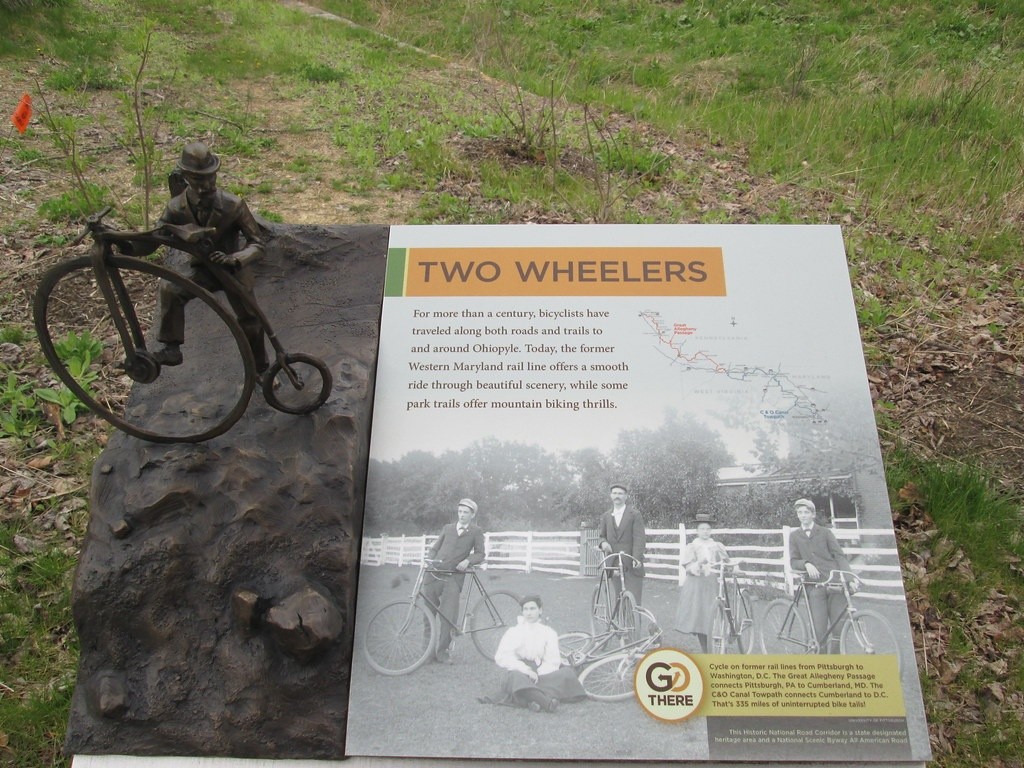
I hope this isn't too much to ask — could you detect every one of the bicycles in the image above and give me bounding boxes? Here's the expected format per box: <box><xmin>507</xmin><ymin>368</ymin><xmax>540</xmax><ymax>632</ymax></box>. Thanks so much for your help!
<box><xmin>590</xmin><ymin>553</ymin><xmax>641</xmax><ymax>650</ymax></box>
<box><xmin>705</xmin><ymin>559</ymin><xmax>754</xmax><ymax>654</ymax></box>
<box><xmin>362</xmin><ymin>559</ymin><xmax>523</xmax><ymax>677</ymax></box>
<box><xmin>558</xmin><ymin>605</ymin><xmax>665</xmax><ymax>702</ymax></box>
<box><xmin>759</xmin><ymin>570</ymin><xmax>900</xmax><ymax>655</ymax></box>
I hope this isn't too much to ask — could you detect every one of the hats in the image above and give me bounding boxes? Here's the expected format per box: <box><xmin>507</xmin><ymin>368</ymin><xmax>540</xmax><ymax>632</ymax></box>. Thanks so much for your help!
<box><xmin>519</xmin><ymin>594</ymin><xmax>541</xmax><ymax>605</ymax></box>
<box><xmin>693</xmin><ymin>514</ymin><xmax>715</xmax><ymax>523</ymax></box>
<box><xmin>795</xmin><ymin>498</ymin><xmax>815</xmax><ymax>510</ymax></box>
<box><xmin>457</xmin><ymin>498</ymin><xmax>478</xmax><ymax>515</ymax></box>
<box><xmin>610</xmin><ymin>483</ymin><xmax>628</xmax><ymax>490</ymax></box>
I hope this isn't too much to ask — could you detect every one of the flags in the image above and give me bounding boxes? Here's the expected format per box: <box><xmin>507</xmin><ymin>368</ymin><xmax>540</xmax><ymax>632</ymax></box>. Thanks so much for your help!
<box><xmin>8</xmin><ymin>95</ymin><xmax>35</xmax><ymax>134</ymax></box>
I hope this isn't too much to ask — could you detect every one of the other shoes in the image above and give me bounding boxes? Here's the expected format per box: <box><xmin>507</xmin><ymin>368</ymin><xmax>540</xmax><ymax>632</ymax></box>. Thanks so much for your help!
<box><xmin>420</xmin><ymin>650</ymin><xmax>452</xmax><ymax>664</ymax></box>
<box><xmin>528</xmin><ymin>698</ymin><xmax>558</xmax><ymax>713</ymax></box>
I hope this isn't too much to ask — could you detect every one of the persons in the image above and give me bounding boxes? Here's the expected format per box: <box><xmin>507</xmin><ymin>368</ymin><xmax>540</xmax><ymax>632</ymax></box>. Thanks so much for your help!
<box><xmin>422</xmin><ymin>498</ymin><xmax>485</xmax><ymax>666</ymax></box>
<box><xmin>111</xmin><ymin>141</ymin><xmax>271</xmax><ymax>376</ymax></box>
<box><xmin>493</xmin><ymin>594</ymin><xmax>562</xmax><ymax>713</ymax></box>
<box><xmin>597</xmin><ymin>484</ymin><xmax>646</xmax><ymax>636</ymax></box>
<box><xmin>674</xmin><ymin>513</ymin><xmax>731</xmax><ymax>655</ymax></box>
<box><xmin>788</xmin><ymin>499</ymin><xmax>860</xmax><ymax>654</ymax></box>
